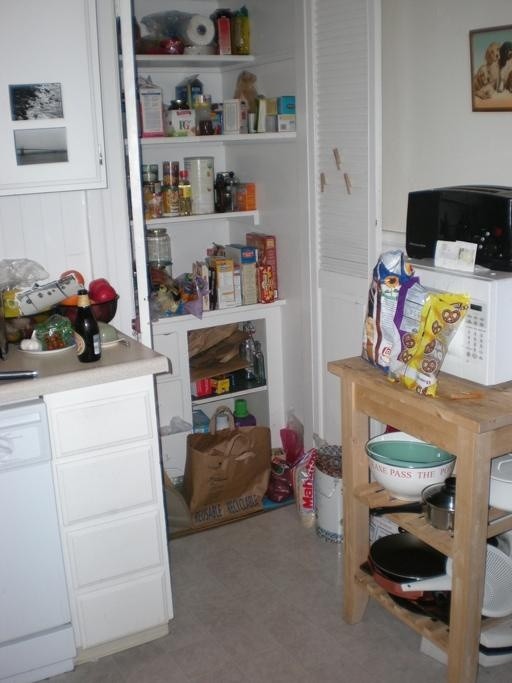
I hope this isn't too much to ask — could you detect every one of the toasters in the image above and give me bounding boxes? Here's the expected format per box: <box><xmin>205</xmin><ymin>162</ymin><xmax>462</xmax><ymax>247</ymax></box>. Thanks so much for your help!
<box><xmin>404</xmin><ymin>185</ymin><xmax>512</xmax><ymax>270</ymax></box>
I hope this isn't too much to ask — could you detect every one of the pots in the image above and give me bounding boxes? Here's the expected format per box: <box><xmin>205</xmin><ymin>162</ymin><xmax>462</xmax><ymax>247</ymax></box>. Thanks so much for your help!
<box><xmin>359</xmin><ymin>477</ymin><xmax>455</xmax><ymax>600</ymax></box>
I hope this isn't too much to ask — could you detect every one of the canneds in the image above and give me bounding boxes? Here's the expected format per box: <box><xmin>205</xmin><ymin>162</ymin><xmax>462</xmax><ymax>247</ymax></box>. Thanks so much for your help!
<box><xmin>167</xmin><ymin>98</ymin><xmax>190</xmax><ymax>110</ymax></box>
<box><xmin>163</xmin><ymin>162</ymin><xmax>178</xmax><ymax>187</ymax></box>
<box><xmin>161</xmin><ymin>186</ymin><xmax>178</xmax><ymax>216</ymax></box>
<box><xmin>143</xmin><ymin>184</ymin><xmax>162</xmax><ymax>218</ymax></box>
<box><xmin>142</xmin><ymin>165</ymin><xmax>158</xmax><ymax>182</ymax></box>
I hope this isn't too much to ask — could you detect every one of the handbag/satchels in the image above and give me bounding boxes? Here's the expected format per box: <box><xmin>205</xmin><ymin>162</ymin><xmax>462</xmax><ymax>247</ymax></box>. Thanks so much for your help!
<box><xmin>185</xmin><ymin>407</ymin><xmax>271</xmax><ymax>530</ymax></box>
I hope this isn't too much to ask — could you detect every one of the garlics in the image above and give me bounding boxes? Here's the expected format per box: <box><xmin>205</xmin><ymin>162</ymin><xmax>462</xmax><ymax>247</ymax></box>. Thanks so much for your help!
<box><xmin>21</xmin><ymin>330</ymin><xmax>42</xmax><ymax>352</ymax></box>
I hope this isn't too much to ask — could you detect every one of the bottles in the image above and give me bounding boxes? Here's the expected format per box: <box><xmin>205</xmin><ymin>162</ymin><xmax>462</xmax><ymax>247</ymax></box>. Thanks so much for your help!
<box><xmin>232</xmin><ymin>399</ymin><xmax>256</xmax><ymax>428</ymax></box>
<box><xmin>225</xmin><ymin>176</ymin><xmax>240</xmax><ymax>212</ymax></box>
<box><xmin>243</xmin><ymin>325</ymin><xmax>265</xmax><ymax>385</ymax></box>
<box><xmin>72</xmin><ymin>290</ymin><xmax>102</xmax><ymax>362</ymax></box>
<box><xmin>178</xmin><ymin>171</ymin><xmax>192</xmax><ymax>216</ymax></box>
<box><xmin>145</xmin><ymin>227</ymin><xmax>172</xmax><ymax>281</ymax></box>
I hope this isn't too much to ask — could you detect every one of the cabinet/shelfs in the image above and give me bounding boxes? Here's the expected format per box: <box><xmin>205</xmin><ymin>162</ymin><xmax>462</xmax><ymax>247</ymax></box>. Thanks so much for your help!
<box><xmin>40</xmin><ymin>375</ymin><xmax>173</xmax><ymax>667</ymax></box>
<box><xmin>95</xmin><ymin>0</ymin><xmax>311</xmax><ymax>538</ymax></box>
<box><xmin>327</xmin><ymin>355</ymin><xmax>512</xmax><ymax>683</ymax></box>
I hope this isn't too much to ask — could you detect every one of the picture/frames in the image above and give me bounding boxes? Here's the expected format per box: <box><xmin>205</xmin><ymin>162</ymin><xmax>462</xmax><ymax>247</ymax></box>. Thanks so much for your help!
<box><xmin>469</xmin><ymin>25</ymin><xmax>512</xmax><ymax>111</ymax></box>
<box><xmin>0</xmin><ymin>0</ymin><xmax>108</xmax><ymax>198</ymax></box>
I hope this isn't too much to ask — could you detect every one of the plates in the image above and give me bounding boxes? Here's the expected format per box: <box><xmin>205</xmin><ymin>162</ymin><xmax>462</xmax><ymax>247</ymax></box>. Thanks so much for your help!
<box><xmin>99</xmin><ymin>338</ymin><xmax>125</xmax><ymax>348</ymax></box>
<box><xmin>15</xmin><ymin>345</ymin><xmax>74</xmax><ymax>355</ymax></box>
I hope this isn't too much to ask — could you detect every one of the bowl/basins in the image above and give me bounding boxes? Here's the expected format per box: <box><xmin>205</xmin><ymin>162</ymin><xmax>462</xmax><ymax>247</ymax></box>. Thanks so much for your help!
<box><xmin>59</xmin><ymin>296</ymin><xmax>120</xmax><ymax>324</ymax></box>
<box><xmin>364</xmin><ymin>432</ymin><xmax>456</xmax><ymax>502</ymax></box>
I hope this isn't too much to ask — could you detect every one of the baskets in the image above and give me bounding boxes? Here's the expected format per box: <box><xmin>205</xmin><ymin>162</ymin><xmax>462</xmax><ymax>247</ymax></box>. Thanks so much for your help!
<box><xmin>447</xmin><ymin>541</ymin><xmax>512</xmax><ymax>618</ymax></box>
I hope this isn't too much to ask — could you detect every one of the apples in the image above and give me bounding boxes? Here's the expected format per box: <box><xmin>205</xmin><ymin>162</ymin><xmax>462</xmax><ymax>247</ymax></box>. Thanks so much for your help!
<box><xmin>89</xmin><ymin>278</ymin><xmax>108</xmax><ymax>297</ymax></box>
<box><xmin>94</xmin><ymin>284</ymin><xmax>116</xmax><ymax>303</ymax></box>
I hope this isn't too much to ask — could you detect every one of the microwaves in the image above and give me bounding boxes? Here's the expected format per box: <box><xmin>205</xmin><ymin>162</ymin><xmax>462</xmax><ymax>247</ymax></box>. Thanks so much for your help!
<box><xmin>386</xmin><ymin>258</ymin><xmax>511</xmax><ymax>386</ymax></box>
